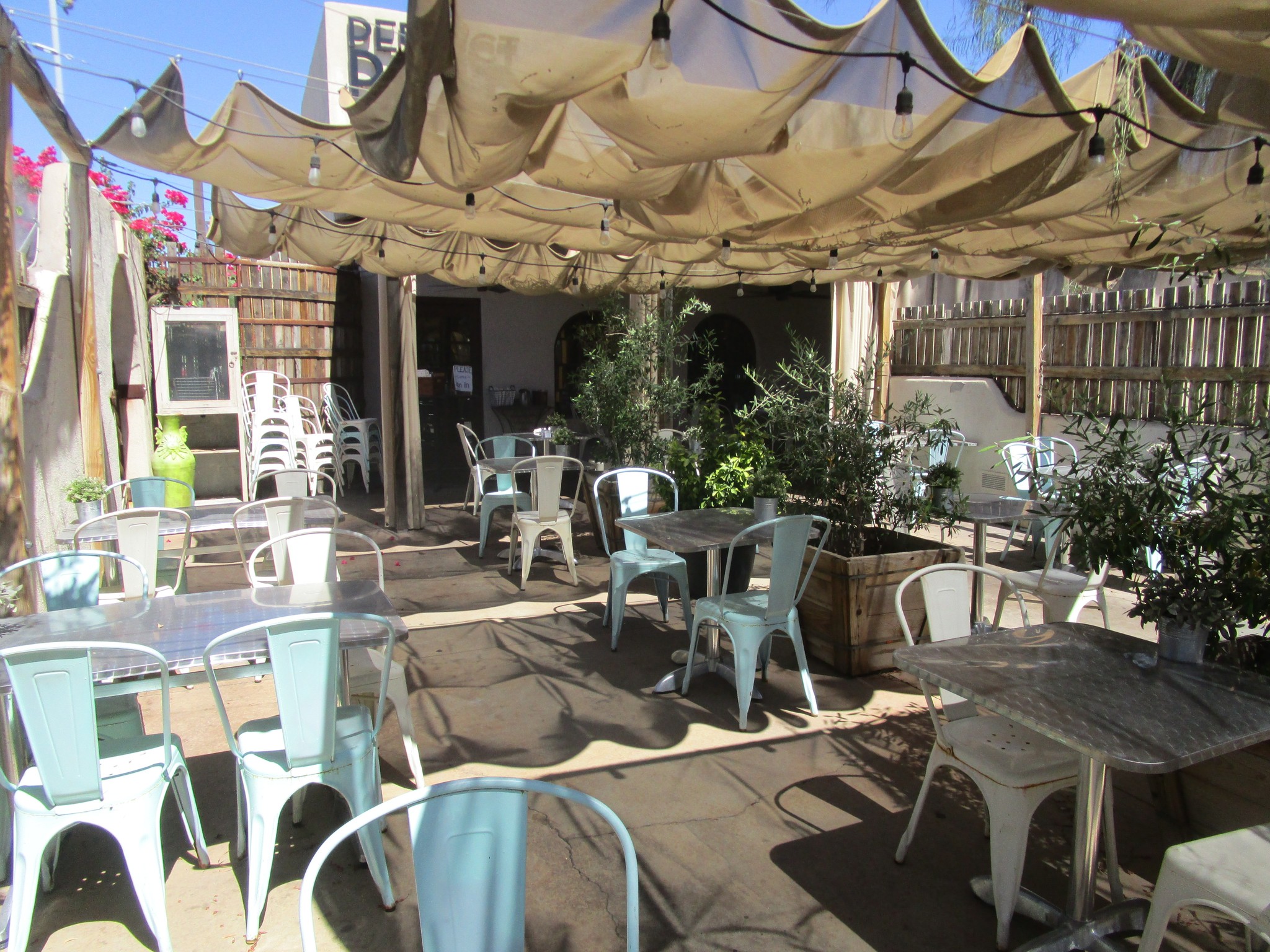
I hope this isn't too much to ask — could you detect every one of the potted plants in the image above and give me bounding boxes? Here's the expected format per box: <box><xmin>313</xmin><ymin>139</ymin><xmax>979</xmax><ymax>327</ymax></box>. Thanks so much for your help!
<box><xmin>650</xmin><ymin>390</ymin><xmax>798</xmax><ymax>601</ymax></box>
<box><xmin>730</xmin><ymin>319</ymin><xmax>967</xmax><ymax>677</ymax></box>
<box><xmin>546</xmin><ymin>412</ymin><xmax>567</xmax><ymax>431</ymax></box>
<box><xmin>552</xmin><ymin>428</ymin><xmax>574</xmax><ymax>458</ymax></box>
<box><xmin>1130</xmin><ymin>572</ymin><xmax>1242</xmax><ymax>664</ymax></box>
<box><xmin>64</xmin><ymin>474</ymin><xmax>111</xmax><ymax>524</ymax></box>
<box><xmin>919</xmin><ymin>460</ymin><xmax>964</xmax><ymax>510</ymax></box>
<box><xmin>743</xmin><ymin>466</ymin><xmax>792</xmax><ymax>520</ymax></box>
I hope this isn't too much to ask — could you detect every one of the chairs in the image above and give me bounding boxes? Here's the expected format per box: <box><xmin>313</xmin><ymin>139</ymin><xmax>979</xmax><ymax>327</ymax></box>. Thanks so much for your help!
<box><xmin>0</xmin><ymin>369</ymin><xmax>425</xmax><ymax>952</ymax></box>
<box><xmin>300</xmin><ymin>777</ymin><xmax>640</xmax><ymax>952</ymax></box>
<box><xmin>455</xmin><ymin>422</ymin><xmax>1270</xmax><ymax>952</ymax></box>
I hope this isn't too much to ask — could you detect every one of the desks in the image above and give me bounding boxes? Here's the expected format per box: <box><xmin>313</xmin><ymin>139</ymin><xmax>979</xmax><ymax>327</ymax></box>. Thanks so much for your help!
<box><xmin>885</xmin><ymin>494</ymin><xmax>1075</xmax><ymax>623</ymax></box>
<box><xmin>1017</xmin><ymin>462</ymin><xmax>1150</xmax><ymax>570</ymax></box>
<box><xmin>891</xmin><ymin>622</ymin><xmax>1270</xmax><ymax>952</ymax></box>
<box><xmin>477</xmin><ymin>458</ymin><xmax>596</xmax><ymax>569</ymax></box>
<box><xmin>0</xmin><ymin>579</ymin><xmax>409</xmax><ymax>825</ymax></box>
<box><xmin>55</xmin><ymin>495</ymin><xmax>345</xmax><ymax>586</ymax></box>
<box><xmin>506</xmin><ymin>432</ymin><xmax>589</xmax><ymax>456</ymax></box>
<box><xmin>614</xmin><ymin>506</ymin><xmax>820</xmax><ymax>701</ymax></box>
<box><xmin>914</xmin><ymin>437</ymin><xmax>977</xmax><ymax>448</ymax></box>
